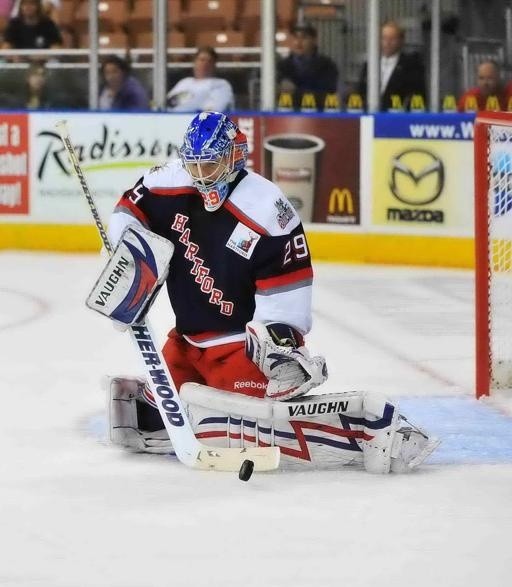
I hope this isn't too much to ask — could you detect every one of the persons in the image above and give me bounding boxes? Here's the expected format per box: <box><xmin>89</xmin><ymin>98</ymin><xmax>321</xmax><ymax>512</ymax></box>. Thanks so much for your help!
<box><xmin>457</xmin><ymin>61</ymin><xmax>511</xmax><ymax>110</ymax></box>
<box><xmin>11</xmin><ymin>64</ymin><xmax>58</xmax><ymax>111</ymax></box>
<box><xmin>98</xmin><ymin>54</ymin><xmax>149</xmax><ymax>110</ymax></box>
<box><xmin>356</xmin><ymin>24</ymin><xmax>426</xmax><ymax>111</ymax></box>
<box><xmin>101</xmin><ymin>110</ymin><xmax>441</xmax><ymax>471</ymax></box>
<box><xmin>276</xmin><ymin>24</ymin><xmax>338</xmax><ymax>105</ymax></box>
<box><xmin>167</xmin><ymin>47</ymin><xmax>236</xmax><ymax>114</ymax></box>
<box><xmin>3</xmin><ymin>0</ymin><xmax>62</xmax><ymax>59</ymax></box>
<box><xmin>11</xmin><ymin>1</ymin><xmax>58</xmax><ymax>22</ymax></box>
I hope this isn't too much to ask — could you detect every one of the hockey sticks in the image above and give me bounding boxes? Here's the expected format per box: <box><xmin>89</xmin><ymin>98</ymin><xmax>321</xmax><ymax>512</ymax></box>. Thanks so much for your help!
<box><xmin>54</xmin><ymin>119</ymin><xmax>280</xmax><ymax>472</ymax></box>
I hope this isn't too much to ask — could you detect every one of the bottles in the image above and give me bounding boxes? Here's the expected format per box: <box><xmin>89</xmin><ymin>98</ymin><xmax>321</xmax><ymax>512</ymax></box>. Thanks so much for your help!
<box><xmin>278</xmin><ymin>80</ymin><xmax>291</xmax><ymax>95</ymax></box>
<box><xmin>248</xmin><ymin>70</ymin><xmax>260</xmax><ymax>101</ymax></box>
<box><xmin>100</xmin><ymin>85</ymin><xmax>112</xmax><ymax>111</ymax></box>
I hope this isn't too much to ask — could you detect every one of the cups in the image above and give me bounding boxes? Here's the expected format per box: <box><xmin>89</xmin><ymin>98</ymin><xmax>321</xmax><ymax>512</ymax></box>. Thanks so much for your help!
<box><xmin>263</xmin><ymin>131</ymin><xmax>327</xmax><ymax>224</ymax></box>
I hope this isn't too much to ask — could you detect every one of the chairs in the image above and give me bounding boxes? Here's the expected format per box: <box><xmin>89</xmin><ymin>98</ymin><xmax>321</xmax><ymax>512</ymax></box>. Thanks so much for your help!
<box><xmin>249</xmin><ymin>78</ymin><xmax>260</xmax><ymax>111</ymax></box>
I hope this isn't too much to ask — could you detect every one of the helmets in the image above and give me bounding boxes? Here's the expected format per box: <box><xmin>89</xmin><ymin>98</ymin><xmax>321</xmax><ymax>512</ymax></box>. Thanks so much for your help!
<box><xmin>179</xmin><ymin>112</ymin><xmax>248</xmax><ymax>213</ymax></box>
